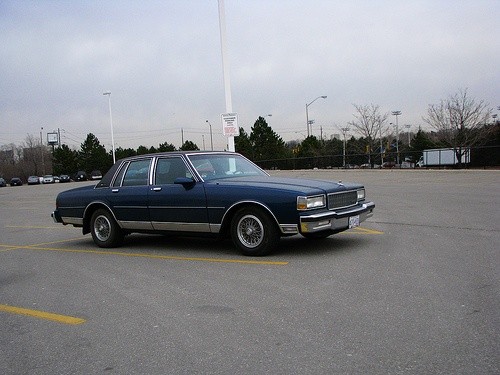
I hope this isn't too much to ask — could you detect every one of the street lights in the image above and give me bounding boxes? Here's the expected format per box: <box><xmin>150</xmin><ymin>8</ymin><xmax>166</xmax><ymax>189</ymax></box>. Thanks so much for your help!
<box><xmin>206</xmin><ymin>120</ymin><xmax>213</xmax><ymax>151</ymax></box>
<box><xmin>103</xmin><ymin>91</ymin><xmax>116</xmax><ymax>165</ymax></box>
<box><xmin>306</xmin><ymin>96</ymin><xmax>328</xmax><ymax>138</ymax></box>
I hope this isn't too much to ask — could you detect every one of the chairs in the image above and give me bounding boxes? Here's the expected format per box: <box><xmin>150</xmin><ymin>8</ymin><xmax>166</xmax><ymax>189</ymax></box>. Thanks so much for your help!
<box><xmin>168</xmin><ymin>162</ymin><xmax>186</xmax><ymax>184</ymax></box>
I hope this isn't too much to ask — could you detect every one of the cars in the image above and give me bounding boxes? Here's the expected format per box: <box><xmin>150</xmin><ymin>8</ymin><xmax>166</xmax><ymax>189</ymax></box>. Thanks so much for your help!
<box><xmin>0</xmin><ymin>177</ymin><xmax>6</xmax><ymax>187</ymax></box>
<box><xmin>50</xmin><ymin>150</ymin><xmax>375</xmax><ymax>258</ymax></box>
<box><xmin>10</xmin><ymin>177</ymin><xmax>23</xmax><ymax>186</ymax></box>
<box><xmin>27</xmin><ymin>170</ymin><xmax>103</xmax><ymax>185</ymax></box>
